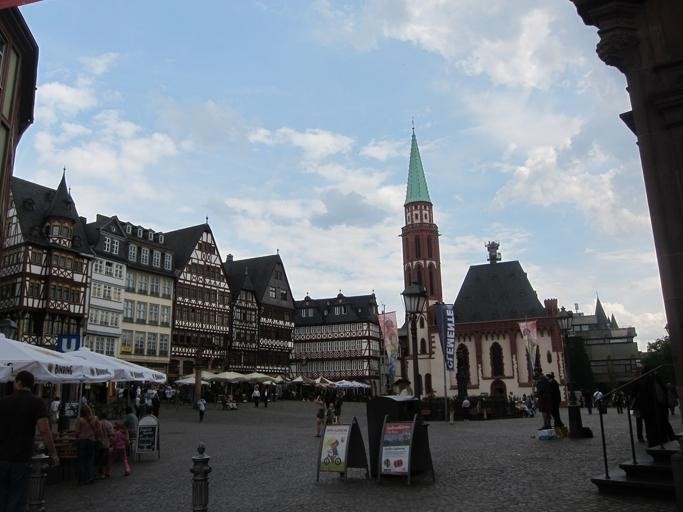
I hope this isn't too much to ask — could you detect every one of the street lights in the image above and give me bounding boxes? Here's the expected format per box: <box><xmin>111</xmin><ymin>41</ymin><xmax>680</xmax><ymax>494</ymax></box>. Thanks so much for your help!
<box><xmin>554</xmin><ymin>305</ymin><xmax>594</xmax><ymax>439</ymax></box>
<box><xmin>400</xmin><ymin>280</ymin><xmax>429</xmax><ymax>399</ymax></box>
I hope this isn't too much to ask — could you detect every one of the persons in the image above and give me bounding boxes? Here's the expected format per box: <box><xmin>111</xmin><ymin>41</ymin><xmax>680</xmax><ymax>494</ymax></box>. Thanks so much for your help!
<box><xmin>462</xmin><ymin>395</ymin><xmax>472</xmax><ymax>422</ymax></box>
<box><xmin>584</xmin><ymin>353</ymin><xmax>680</xmax><ymax>447</ymax></box>
<box><xmin>0</xmin><ymin>371</ymin><xmax>59</xmax><ymax>512</ymax></box>
<box><xmin>509</xmin><ymin>367</ymin><xmax>566</xmax><ymax>430</ymax></box>
<box><xmin>197</xmin><ymin>385</ymin><xmax>370</xmax><ymax>437</ymax></box>
<box><xmin>50</xmin><ymin>382</ymin><xmax>181</xmax><ymax>479</ymax></box>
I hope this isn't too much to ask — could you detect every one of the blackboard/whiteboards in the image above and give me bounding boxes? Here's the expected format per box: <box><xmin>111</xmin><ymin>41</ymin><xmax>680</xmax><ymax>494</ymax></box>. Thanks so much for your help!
<box><xmin>136</xmin><ymin>425</ymin><xmax>158</xmax><ymax>454</ymax></box>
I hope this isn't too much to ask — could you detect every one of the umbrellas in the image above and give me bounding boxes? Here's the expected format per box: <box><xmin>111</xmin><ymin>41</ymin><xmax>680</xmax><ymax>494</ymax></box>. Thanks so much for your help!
<box><xmin>0</xmin><ymin>333</ymin><xmax>167</xmax><ymax>415</ymax></box>
<box><xmin>173</xmin><ymin>370</ymin><xmax>371</xmax><ymax>388</ymax></box>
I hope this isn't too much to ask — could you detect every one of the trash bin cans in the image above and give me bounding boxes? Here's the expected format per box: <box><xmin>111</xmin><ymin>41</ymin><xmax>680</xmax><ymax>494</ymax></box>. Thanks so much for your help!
<box><xmin>367</xmin><ymin>396</ymin><xmax>421</xmax><ymax>480</ymax></box>
